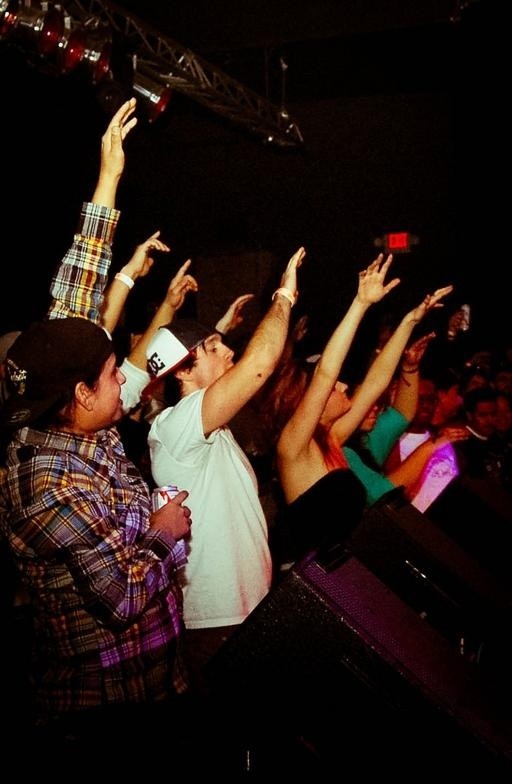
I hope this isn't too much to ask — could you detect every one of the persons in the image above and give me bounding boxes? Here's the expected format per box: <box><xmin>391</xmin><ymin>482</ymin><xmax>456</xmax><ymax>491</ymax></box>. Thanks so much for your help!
<box><xmin>2</xmin><ymin>96</ymin><xmax>193</xmax><ymax>783</ymax></box>
<box><xmin>98</xmin><ymin>230</ymin><xmax>510</xmax><ymax>784</ymax></box>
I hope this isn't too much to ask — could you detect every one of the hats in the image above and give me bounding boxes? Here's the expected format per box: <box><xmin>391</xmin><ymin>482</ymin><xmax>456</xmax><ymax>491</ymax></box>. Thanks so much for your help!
<box><xmin>0</xmin><ymin>316</ymin><xmax>116</xmax><ymax>432</ymax></box>
<box><xmin>141</xmin><ymin>315</ymin><xmax>226</xmax><ymax>400</ymax></box>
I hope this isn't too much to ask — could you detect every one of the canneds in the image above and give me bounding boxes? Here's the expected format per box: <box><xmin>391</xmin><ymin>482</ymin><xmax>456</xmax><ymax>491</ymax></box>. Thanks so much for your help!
<box><xmin>459</xmin><ymin>304</ymin><xmax>470</xmax><ymax>330</ymax></box>
<box><xmin>152</xmin><ymin>485</ymin><xmax>186</xmax><ymax>555</ymax></box>
